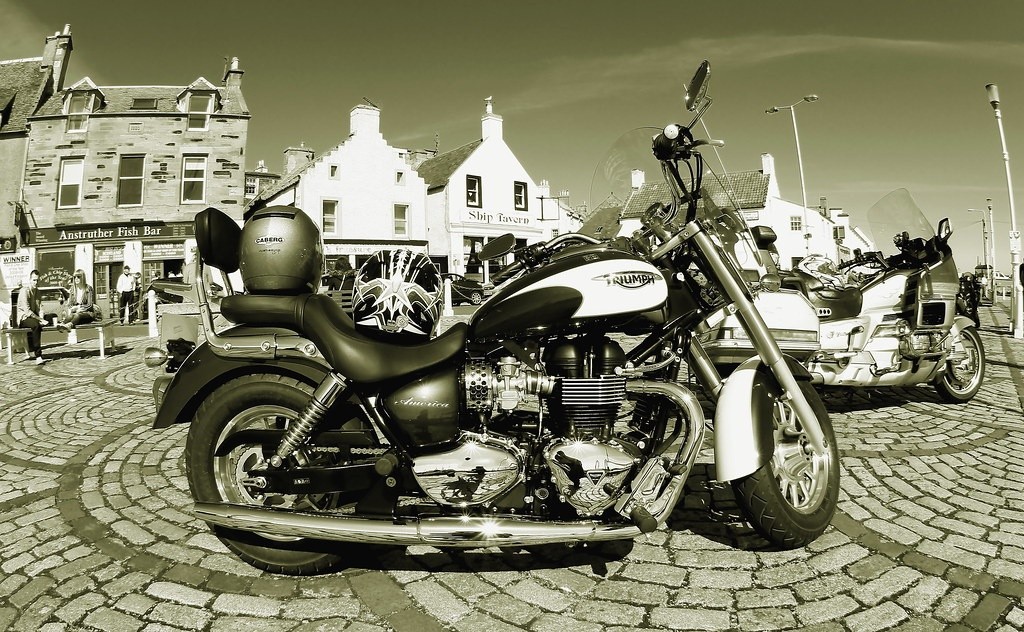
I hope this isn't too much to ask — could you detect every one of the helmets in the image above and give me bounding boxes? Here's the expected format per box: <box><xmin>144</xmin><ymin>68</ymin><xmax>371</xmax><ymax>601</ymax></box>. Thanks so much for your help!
<box><xmin>239</xmin><ymin>205</ymin><xmax>323</xmax><ymax>294</ymax></box>
<box><xmin>798</xmin><ymin>254</ymin><xmax>845</xmax><ymax>293</ymax></box>
<box><xmin>351</xmin><ymin>249</ymin><xmax>443</xmax><ymax>346</ymax></box>
<box><xmin>962</xmin><ymin>272</ymin><xmax>973</xmax><ymax>282</ymax></box>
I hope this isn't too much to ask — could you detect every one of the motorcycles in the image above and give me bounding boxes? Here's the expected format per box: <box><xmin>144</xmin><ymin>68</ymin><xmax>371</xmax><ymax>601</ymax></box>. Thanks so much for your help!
<box><xmin>693</xmin><ymin>189</ymin><xmax>986</xmax><ymax>400</ymax></box>
<box><xmin>146</xmin><ymin>60</ymin><xmax>841</xmax><ymax>576</ymax></box>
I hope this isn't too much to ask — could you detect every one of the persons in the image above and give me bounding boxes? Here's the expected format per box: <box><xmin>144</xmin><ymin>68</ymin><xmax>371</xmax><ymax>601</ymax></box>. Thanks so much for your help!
<box><xmin>329</xmin><ymin>258</ymin><xmax>356</xmax><ymax>320</ymax></box>
<box><xmin>119</xmin><ymin>265</ymin><xmax>183</xmax><ymax>325</ymax></box>
<box><xmin>16</xmin><ymin>269</ymin><xmax>48</xmax><ymax>366</ymax></box>
<box><xmin>57</xmin><ymin>269</ymin><xmax>95</xmax><ymax>332</ymax></box>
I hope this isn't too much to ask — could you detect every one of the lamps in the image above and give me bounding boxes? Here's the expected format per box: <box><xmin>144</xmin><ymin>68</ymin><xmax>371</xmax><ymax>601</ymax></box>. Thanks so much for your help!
<box><xmin>7</xmin><ymin>199</ymin><xmax>28</xmax><ymax>206</ymax></box>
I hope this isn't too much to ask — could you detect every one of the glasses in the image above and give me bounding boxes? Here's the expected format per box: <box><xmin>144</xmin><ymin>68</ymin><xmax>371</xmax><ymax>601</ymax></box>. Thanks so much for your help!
<box><xmin>74</xmin><ymin>276</ymin><xmax>81</xmax><ymax>278</ymax></box>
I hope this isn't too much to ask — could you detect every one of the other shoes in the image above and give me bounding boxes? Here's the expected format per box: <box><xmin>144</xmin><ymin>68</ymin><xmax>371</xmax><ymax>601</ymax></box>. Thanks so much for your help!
<box><xmin>129</xmin><ymin>323</ymin><xmax>134</xmax><ymax>326</ymax></box>
<box><xmin>57</xmin><ymin>324</ymin><xmax>64</xmax><ymax>333</ymax></box>
<box><xmin>120</xmin><ymin>323</ymin><xmax>124</xmax><ymax>326</ymax></box>
<box><xmin>61</xmin><ymin>324</ymin><xmax>71</xmax><ymax>333</ymax></box>
<box><xmin>36</xmin><ymin>357</ymin><xmax>44</xmax><ymax>365</ymax></box>
<box><xmin>28</xmin><ymin>351</ymin><xmax>36</xmax><ymax>358</ymax></box>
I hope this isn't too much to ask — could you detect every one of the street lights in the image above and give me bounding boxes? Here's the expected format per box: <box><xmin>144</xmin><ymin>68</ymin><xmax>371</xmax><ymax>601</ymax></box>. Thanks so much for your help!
<box><xmin>764</xmin><ymin>94</ymin><xmax>818</xmax><ymax>208</ymax></box>
<box><xmin>986</xmin><ymin>83</ymin><xmax>1024</xmax><ymax>340</ymax></box>
<box><xmin>986</xmin><ymin>199</ymin><xmax>994</xmax><ymax>298</ymax></box>
<box><xmin>968</xmin><ymin>209</ymin><xmax>989</xmax><ymax>282</ymax></box>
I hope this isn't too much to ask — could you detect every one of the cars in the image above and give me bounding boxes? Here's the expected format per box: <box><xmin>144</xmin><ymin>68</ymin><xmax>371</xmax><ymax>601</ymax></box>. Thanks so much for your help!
<box><xmin>483</xmin><ymin>282</ymin><xmax>495</xmax><ymax>295</ymax></box>
<box><xmin>439</xmin><ymin>273</ymin><xmax>484</xmax><ymax>305</ymax></box>
<box><xmin>34</xmin><ymin>286</ymin><xmax>104</xmax><ymax>325</ymax></box>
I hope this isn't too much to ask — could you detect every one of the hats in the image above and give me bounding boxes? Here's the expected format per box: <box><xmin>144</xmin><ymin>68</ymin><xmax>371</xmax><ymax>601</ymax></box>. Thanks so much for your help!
<box><xmin>124</xmin><ymin>266</ymin><xmax>130</xmax><ymax>269</ymax></box>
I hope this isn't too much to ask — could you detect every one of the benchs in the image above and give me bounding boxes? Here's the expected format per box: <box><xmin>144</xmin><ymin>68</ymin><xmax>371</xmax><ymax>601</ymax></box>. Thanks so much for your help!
<box><xmin>2</xmin><ymin>318</ymin><xmax>118</xmax><ymax>364</ymax></box>
<box><xmin>324</xmin><ymin>290</ymin><xmax>352</xmax><ymax>314</ymax></box>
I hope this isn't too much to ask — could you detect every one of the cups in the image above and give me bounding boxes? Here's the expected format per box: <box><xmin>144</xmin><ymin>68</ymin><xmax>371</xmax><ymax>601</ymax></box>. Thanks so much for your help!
<box><xmin>52</xmin><ymin>316</ymin><xmax>58</xmax><ymax>327</ymax></box>
<box><xmin>38</xmin><ymin>311</ymin><xmax>45</xmax><ymax>318</ymax></box>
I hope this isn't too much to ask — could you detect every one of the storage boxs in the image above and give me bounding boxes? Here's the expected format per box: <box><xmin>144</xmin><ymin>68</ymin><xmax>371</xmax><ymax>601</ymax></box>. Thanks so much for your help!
<box><xmin>707</xmin><ymin>288</ymin><xmax>820</xmax><ymax>364</ymax></box>
